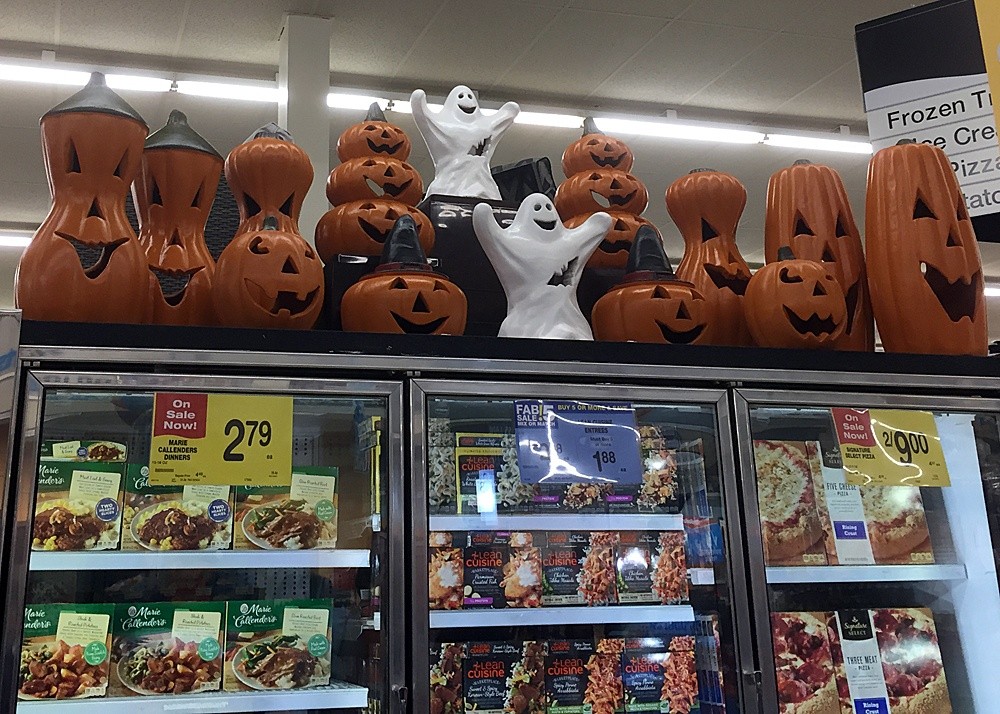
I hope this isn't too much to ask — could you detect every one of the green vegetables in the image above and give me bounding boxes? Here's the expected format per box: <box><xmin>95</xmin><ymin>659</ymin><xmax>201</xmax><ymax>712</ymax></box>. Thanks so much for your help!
<box><xmin>20</xmin><ymin>644</ymin><xmax>53</xmax><ymax>681</ymax></box>
<box><xmin>125</xmin><ymin>641</ymin><xmax>168</xmax><ymax>684</ymax></box>
<box><xmin>241</xmin><ymin>634</ymin><xmax>300</xmax><ymax>667</ymax></box>
<box><xmin>248</xmin><ymin>499</ymin><xmax>308</xmax><ymax>530</ymax></box>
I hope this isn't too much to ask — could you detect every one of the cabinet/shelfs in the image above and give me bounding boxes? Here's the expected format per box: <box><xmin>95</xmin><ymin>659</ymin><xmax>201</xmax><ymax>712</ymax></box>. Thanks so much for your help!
<box><xmin>0</xmin><ymin>318</ymin><xmax>1000</xmax><ymax>714</ymax></box>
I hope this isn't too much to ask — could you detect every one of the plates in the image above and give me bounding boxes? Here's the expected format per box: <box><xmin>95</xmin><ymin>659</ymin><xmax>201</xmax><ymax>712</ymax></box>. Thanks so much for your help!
<box><xmin>118</xmin><ymin>640</ymin><xmax>200</xmax><ymax>695</ymax></box>
<box><xmin>242</xmin><ymin>500</ymin><xmax>319</xmax><ymax>549</ymax></box>
<box><xmin>17</xmin><ymin>642</ymin><xmax>108</xmax><ymax>701</ymax></box>
<box><xmin>30</xmin><ymin>499</ymin><xmax>97</xmax><ymax>551</ymax></box>
<box><xmin>232</xmin><ymin>635</ymin><xmax>314</xmax><ymax>689</ymax></box>
<box><xmin>130</xmin><ymin>500</ymin><xmax>213</xmax><ymax>550</ymax></box>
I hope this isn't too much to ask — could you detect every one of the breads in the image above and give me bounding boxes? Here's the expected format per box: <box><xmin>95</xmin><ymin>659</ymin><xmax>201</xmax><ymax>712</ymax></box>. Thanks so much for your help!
<box><xmin>755</xmin><ymin>439</ymin><xmax>954</xmax><ymax>714</ymax></box>
